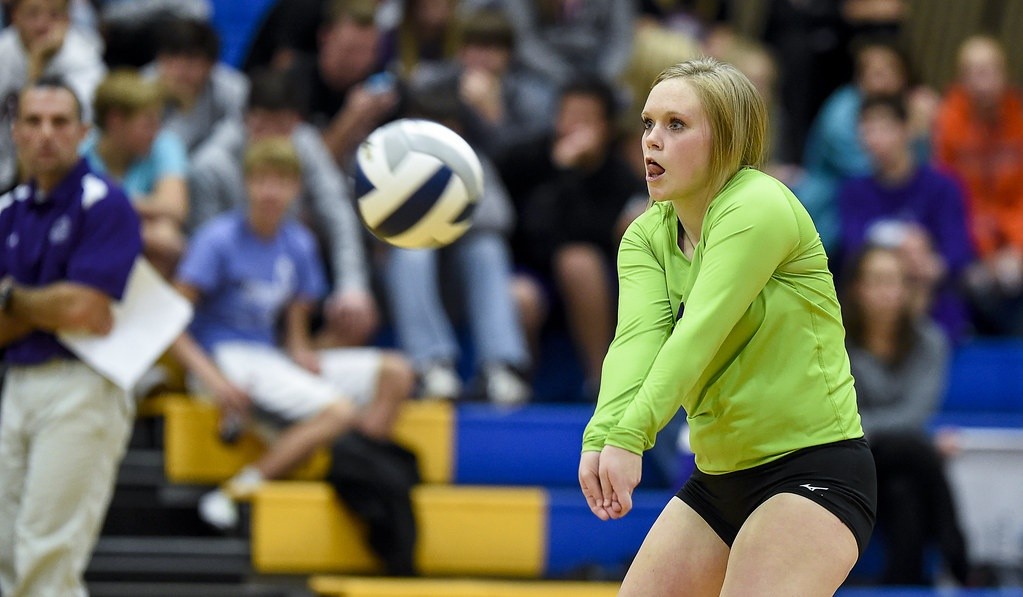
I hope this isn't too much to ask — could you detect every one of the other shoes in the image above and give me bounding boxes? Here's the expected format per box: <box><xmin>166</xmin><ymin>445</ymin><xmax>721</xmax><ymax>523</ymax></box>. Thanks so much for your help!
<box><xmin>200</xmin><ymin>491</ymin><xmax>240</xmax><ymax>529</ymax></box>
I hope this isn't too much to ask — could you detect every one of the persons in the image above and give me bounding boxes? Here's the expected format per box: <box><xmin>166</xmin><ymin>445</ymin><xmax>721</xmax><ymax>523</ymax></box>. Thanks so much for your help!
<box><xmin>0</xmin><ymin>2</ymin><xmax>1023</xmax><ymax>595</ymax></box>
<box><xmin>577</xmin><ymin>60</ymin><xmax>877</xmax><ymax>595</ymax></box>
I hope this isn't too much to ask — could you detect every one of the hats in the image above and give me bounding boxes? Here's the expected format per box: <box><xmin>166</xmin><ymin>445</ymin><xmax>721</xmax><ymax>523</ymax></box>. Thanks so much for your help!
<box><xmin>244</xmin><ymin>135</ymin><xmax>297</xmax><ymax>174</ymax></box>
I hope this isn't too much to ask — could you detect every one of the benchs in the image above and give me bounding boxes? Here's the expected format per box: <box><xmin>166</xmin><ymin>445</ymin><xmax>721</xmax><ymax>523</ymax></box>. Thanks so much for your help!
<box><xmin>139</xmin><ymin>337</ymin><xmax>1023</xmax><ymax>597</ymax></box>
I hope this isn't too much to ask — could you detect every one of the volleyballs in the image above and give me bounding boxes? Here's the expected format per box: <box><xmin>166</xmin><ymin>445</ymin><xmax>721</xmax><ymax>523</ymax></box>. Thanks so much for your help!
<box><xmin>348</xmin><ymin>116</ymin><xmax>486</xmax><ymax>252</ymax></box>
